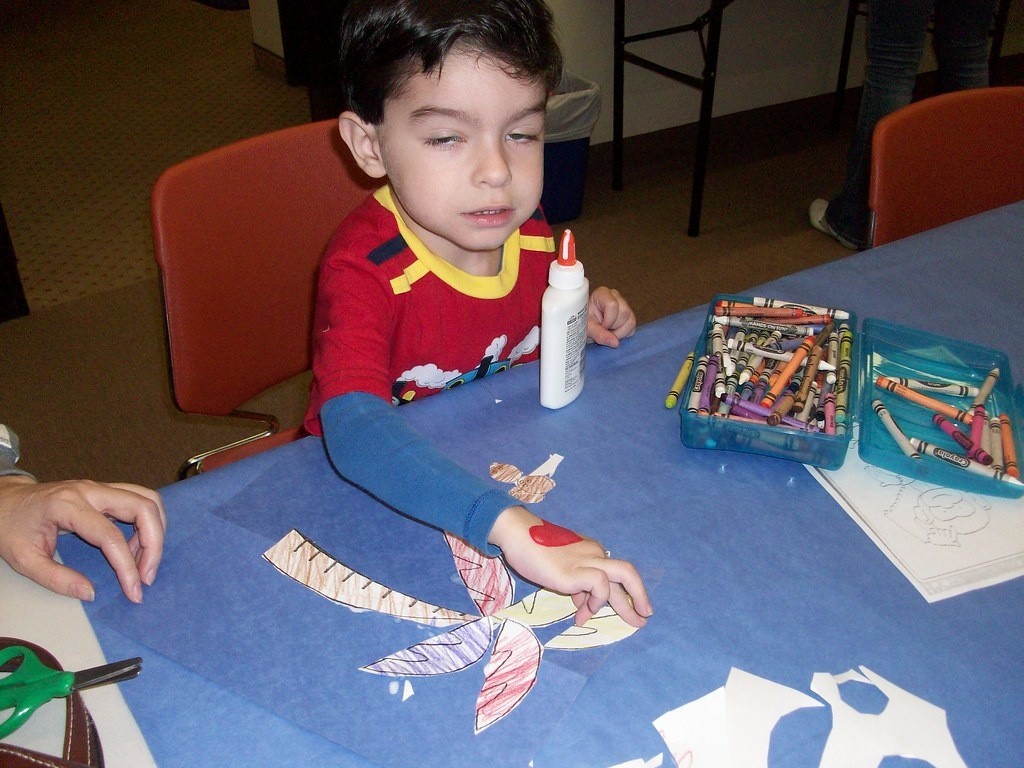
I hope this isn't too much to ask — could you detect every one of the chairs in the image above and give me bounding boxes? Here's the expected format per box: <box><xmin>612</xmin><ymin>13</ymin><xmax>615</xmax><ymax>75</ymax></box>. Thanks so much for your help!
<box><xmin>153</xmin><ymin>114</ymin><xmax>389</xmax><ymax>479</ymax></box>
<box><xmin>865</xmin><ymin>85</ymin><xmax>1024</xmax><ymax>243</ymax></box>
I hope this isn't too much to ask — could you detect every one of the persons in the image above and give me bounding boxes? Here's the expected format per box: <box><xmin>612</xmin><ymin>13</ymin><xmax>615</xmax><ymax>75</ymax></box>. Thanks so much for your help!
<box><xmin>305</xmin><ymin>0</ymin><xmax>653</xmax><ymax>628</ymax></box>
<box><xmin>0</xmin><ymin>424</ymin><xmax>166</xmax><ymax>603</ymax></box>
<box><xmin>807</xmin><ymin>0</ymin><xmax>997</xmax><ymax>250</ymax></box>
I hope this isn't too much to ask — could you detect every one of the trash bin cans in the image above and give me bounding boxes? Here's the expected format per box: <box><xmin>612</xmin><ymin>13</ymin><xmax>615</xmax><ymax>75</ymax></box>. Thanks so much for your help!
<box><xmin>540</xmin><ymin>72</ymin><xmax>603</xmax><ymax>225</ymax></box>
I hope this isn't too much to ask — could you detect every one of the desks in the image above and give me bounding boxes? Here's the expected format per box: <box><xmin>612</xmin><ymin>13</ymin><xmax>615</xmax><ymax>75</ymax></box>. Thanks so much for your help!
<box><xmin>0</xmin><ymin>199</ymin><xmax>1024</xmax><ymax>768</ymax></box>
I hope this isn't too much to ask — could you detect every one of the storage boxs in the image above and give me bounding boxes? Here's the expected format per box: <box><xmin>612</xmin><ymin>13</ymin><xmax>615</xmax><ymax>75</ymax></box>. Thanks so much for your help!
<box><xmin>677</xmin><ymin>291</ymin><xmax>1024</xmax><ymax>500</ymax></box>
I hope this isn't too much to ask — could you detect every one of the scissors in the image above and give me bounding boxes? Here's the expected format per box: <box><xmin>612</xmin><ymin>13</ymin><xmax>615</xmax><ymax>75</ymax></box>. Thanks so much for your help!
<box><xmin>0</xmin><ymin>645</ymin><xmax>143</xmax><ymax>739</ymax></box>
